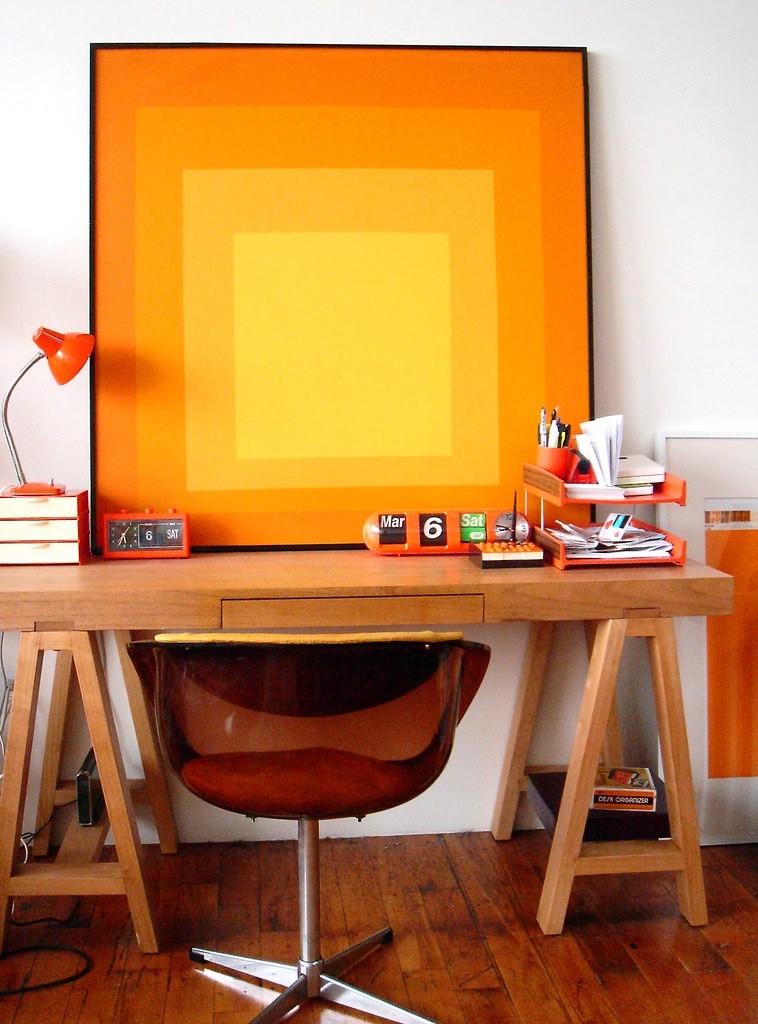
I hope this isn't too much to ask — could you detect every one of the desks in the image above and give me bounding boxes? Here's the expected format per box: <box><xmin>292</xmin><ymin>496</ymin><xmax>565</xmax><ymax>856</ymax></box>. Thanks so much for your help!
<box><xmin>0</xmin><ymin>549</ymin><xmax>733</xmax><ymax>954</ymax></box>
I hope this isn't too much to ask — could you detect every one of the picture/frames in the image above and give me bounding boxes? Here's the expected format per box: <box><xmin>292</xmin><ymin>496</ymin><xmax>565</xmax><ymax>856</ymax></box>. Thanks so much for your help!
<box><xmin>91</xmin><ymin>43</ymin><xmax>594</xmax><ymax>550</ymax></box>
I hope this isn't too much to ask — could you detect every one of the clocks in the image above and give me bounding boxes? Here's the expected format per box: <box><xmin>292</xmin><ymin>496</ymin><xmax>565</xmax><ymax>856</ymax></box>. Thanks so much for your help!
<box><xmin>363</xmin><ymin>508</ymin><xmax>532</xmax><ymax>555</ymax></box>
<box><xmin>106</xmin><ymin>512</ymin><xmax>188</xmax><ymax>560</ymax></box>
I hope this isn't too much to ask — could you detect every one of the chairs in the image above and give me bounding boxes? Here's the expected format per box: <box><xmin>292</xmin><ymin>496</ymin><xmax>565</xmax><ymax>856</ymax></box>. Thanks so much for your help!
<box><xmin>128</xmin><ymin>628</ymin><xmax>490</xmax><ymax>1024</ymax></box>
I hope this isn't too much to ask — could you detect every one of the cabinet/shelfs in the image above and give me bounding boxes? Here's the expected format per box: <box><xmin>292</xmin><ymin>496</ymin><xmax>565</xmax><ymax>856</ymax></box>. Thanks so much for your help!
<box><xmin>524</xmin><ymin>463</ymin><xmax>686</xmax><ymax>570</ymax></box>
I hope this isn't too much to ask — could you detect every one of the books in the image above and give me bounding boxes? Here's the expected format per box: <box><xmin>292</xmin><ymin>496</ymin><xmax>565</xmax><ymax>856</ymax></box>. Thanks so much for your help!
<box><xmin>546</xmin><ymin>413</ymin><xmax>674</xmax><ymax>562</ymax></box>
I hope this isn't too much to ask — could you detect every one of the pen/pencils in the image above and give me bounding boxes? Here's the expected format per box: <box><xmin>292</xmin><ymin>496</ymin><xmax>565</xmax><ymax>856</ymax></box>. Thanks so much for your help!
<box><xmin>537</xmin><ymin>406</ymin><xmax>571</xmax><ymax>448</ymax></box>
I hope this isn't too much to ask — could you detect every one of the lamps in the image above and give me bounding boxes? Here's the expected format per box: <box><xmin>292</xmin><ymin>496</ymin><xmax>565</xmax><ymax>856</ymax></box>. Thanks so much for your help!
<box><xmin>3</xmin><ymin>325</ymin><xmax>94</xmax><ymax>496</ymax></box>
